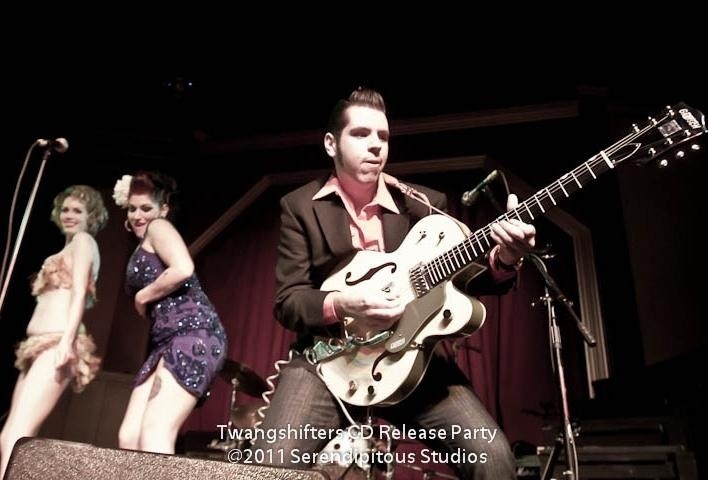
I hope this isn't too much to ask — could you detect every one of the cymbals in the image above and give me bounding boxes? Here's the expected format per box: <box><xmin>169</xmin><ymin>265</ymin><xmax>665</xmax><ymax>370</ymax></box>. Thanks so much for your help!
<box><xmin>225</xmin><ymin>358</ymin><xmax>271</xmax><ymax>398</ymax></box>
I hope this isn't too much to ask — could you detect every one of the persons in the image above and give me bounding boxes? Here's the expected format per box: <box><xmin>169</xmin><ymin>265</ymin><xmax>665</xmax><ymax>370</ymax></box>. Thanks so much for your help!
<box><xmin>112</xmin><ymin>170</ymin><xmax>230</xmax><ymax>456</ymax></box>
<box><xmin>246</xmin><ymin>87</ymin><xmax>536</xmax><ymax>480</ymax></box>
<box><xmin>0</xmin><ymin>184</ymin><xmax>110</xmax><ymax>480</ymax></box>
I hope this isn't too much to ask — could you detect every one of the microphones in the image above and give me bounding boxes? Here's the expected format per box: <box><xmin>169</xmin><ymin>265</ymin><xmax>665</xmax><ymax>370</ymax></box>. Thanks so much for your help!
<box><xmin>38</xmin><ymin>138</ymin><xmax>69</xmax><ymax>153</ymax></box>
<box><xmin>461</xmin><ymin>170</ymin><xmax>498</xmax><ymax>208</ymax></box>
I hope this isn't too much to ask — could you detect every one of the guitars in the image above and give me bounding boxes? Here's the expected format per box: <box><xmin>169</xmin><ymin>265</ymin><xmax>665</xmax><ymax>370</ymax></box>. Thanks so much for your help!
<box><xmin>306</xmin><ymin>102</ymin><xmax>707</xmax><ymax>407</ymax></box>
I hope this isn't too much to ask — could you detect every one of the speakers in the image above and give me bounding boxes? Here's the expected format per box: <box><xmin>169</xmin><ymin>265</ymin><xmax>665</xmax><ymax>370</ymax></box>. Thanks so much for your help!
<box><xmin>4</xmin><ymin>436</ymin><xmax>330</xmax><ymax>480</ymax></box>
